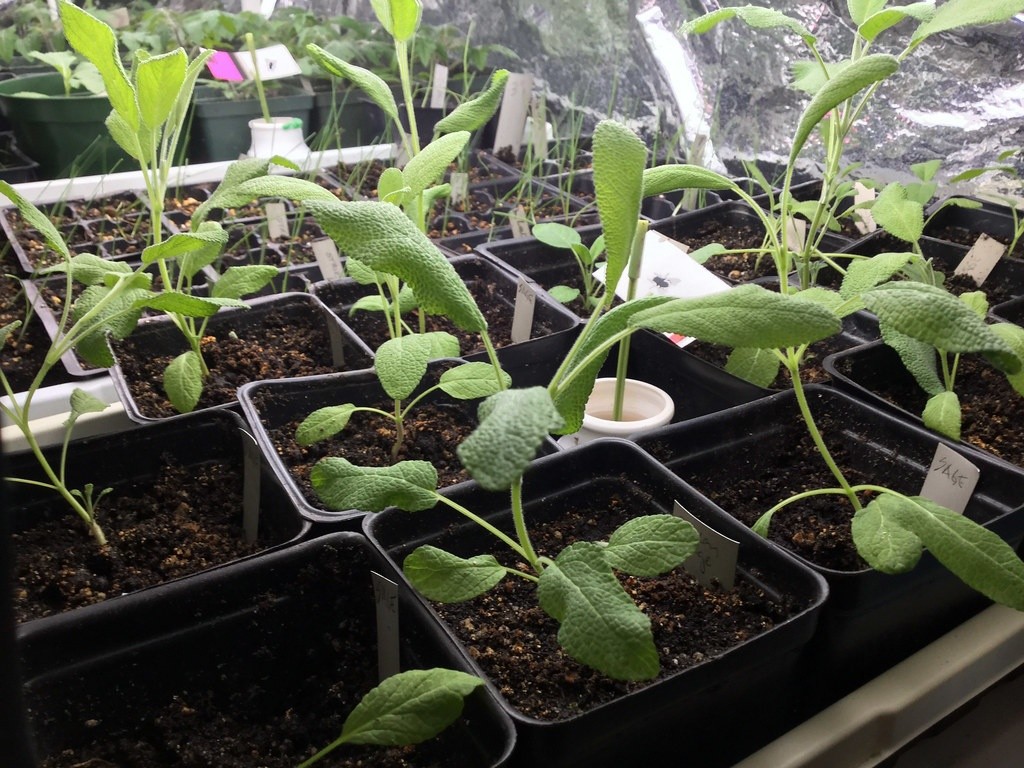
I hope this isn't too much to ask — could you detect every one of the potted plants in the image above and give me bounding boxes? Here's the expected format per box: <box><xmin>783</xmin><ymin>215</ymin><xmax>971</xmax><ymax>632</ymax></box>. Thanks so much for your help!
<box><xmin>0</xmin><ymin>1</ymin><xmax>1024</xmax><ymax>767</ymax></box>
